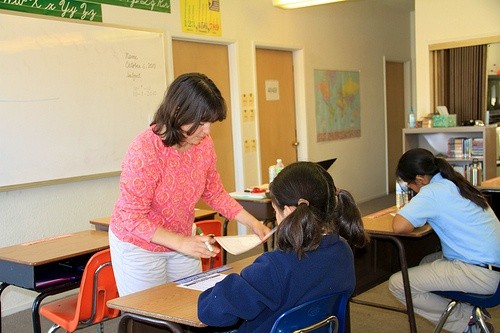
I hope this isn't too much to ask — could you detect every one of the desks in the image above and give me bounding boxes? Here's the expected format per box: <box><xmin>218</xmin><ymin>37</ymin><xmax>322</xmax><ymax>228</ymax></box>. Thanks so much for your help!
<box><xmin>480</xmin><ymin>176</ymin><xmax>500</xmax><ymax>196</ymax></box>
<box><xmin>90</xmin><ymin>208</ymin><xmax>217</xmax><ymax>231</ymax></box>
<box><xmin>346</xmin><ymin>205</ymin><xmax>433</xmax><ymax>333</ymax></box>
<box><xmin>220</xmin><ymin>197</ymin><xmax>275</xmax><ymax>266</ymax></box>
<box><xmin>105</xmin><ymin>251</ymin><xmax>263</xmax><ymax>333</ymax></box>
<box><xmin>0</xmin><ymin>230</ymin><xmax>113</xmax><ymax>333</ymax></box>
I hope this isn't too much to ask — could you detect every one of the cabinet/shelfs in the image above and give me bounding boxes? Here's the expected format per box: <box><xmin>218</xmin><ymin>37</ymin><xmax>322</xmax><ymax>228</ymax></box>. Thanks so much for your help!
<box><xmin>402</xmin><ymin>124</ymin><xmax>497</xmax><ymax>180</ymax></box>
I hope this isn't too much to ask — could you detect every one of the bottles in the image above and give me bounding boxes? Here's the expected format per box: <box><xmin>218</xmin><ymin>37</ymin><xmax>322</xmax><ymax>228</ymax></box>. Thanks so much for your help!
<box><xmin>274</xmin><ymin>158</ymin><xmax>285</xmax><ymax>177</ymax></box>
<box><xmin>408</xmin><ymin>111</ymin><xmax>415</xmax><ymax>128</ymax></box>
<box><xmin>395</xmin><ymin>177</ymin><xmax>409</xmax><ymax>214</ymax></box>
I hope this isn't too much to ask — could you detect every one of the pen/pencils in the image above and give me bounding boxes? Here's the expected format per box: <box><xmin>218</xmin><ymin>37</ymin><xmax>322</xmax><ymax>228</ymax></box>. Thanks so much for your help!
<box><xmin>197</xmin><ymin>226</ymin><xmax>214</xmax><ymax>253</ymax></box>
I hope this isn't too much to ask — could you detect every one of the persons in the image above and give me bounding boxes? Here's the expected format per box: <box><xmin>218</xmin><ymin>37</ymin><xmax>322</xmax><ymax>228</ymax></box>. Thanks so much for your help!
<box><xmin>387</xmin><ymin>148</ymin><xmax>500</xmax><ymax>333</ymax></box>
<box><xmin>109</xmin><ymin>72</ymin><xmax>271</xmax><ymax>333</ymax></box>
<box><xmin>198</xmin><ymin>161</ymin><xmax>370</xmax><ymax>333</ymax></box>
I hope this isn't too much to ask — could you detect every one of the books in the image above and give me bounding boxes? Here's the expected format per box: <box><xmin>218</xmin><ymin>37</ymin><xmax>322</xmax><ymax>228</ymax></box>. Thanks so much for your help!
<box><xmin>448</xmin><ymin>137</ymin><xmax>483</xmax><ymax>158</ymax></box>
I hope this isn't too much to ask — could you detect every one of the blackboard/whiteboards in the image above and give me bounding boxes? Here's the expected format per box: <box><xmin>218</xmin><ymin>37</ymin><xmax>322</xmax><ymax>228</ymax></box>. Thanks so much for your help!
<box><xmin>0</xmin><ymin>6</ymin><xmax>170</xmax><ymax>193</ymax></box>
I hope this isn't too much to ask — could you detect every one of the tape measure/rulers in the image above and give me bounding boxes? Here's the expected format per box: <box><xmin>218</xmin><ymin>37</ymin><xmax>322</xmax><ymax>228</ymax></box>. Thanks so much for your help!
<box><xmin>173</xmin><ymin>264</ymin><xmax>233</xmax><ymax>285</ymax></box>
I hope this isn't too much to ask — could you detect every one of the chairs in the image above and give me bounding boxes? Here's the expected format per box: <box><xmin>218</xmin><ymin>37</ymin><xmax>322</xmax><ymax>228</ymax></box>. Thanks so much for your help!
<box><xmin>193</xmin><ymin>219</ymin><xmax>222</xmax><ymax>271</ymax></box>
<box><xmin>430</xmin><ymin>282</ymin><xmax>500</xmax><ymax>333</ymax></box>
<box><xmin>38</xmin><ymin>247</ymin><xmax>122</xmax><ymax>333</ymax></box>
<box><xmin>269</xmin><ymin>292</ymin><xmax>342</xmax><ymax>333</ymax></box>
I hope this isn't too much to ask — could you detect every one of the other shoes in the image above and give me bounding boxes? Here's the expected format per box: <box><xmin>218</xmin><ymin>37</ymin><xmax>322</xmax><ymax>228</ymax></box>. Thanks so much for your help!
<box><xmin>462</xmin><ymin>309</ymin><xmax>495</xmax><ymax>333</ymax></box>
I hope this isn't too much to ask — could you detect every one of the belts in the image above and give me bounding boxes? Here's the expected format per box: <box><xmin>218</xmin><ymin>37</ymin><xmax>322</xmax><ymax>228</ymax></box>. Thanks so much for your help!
<box><xmin>473</xmin><ymin>262</ymin><xmax>500</xmax><ymax>272</ymax></box>
